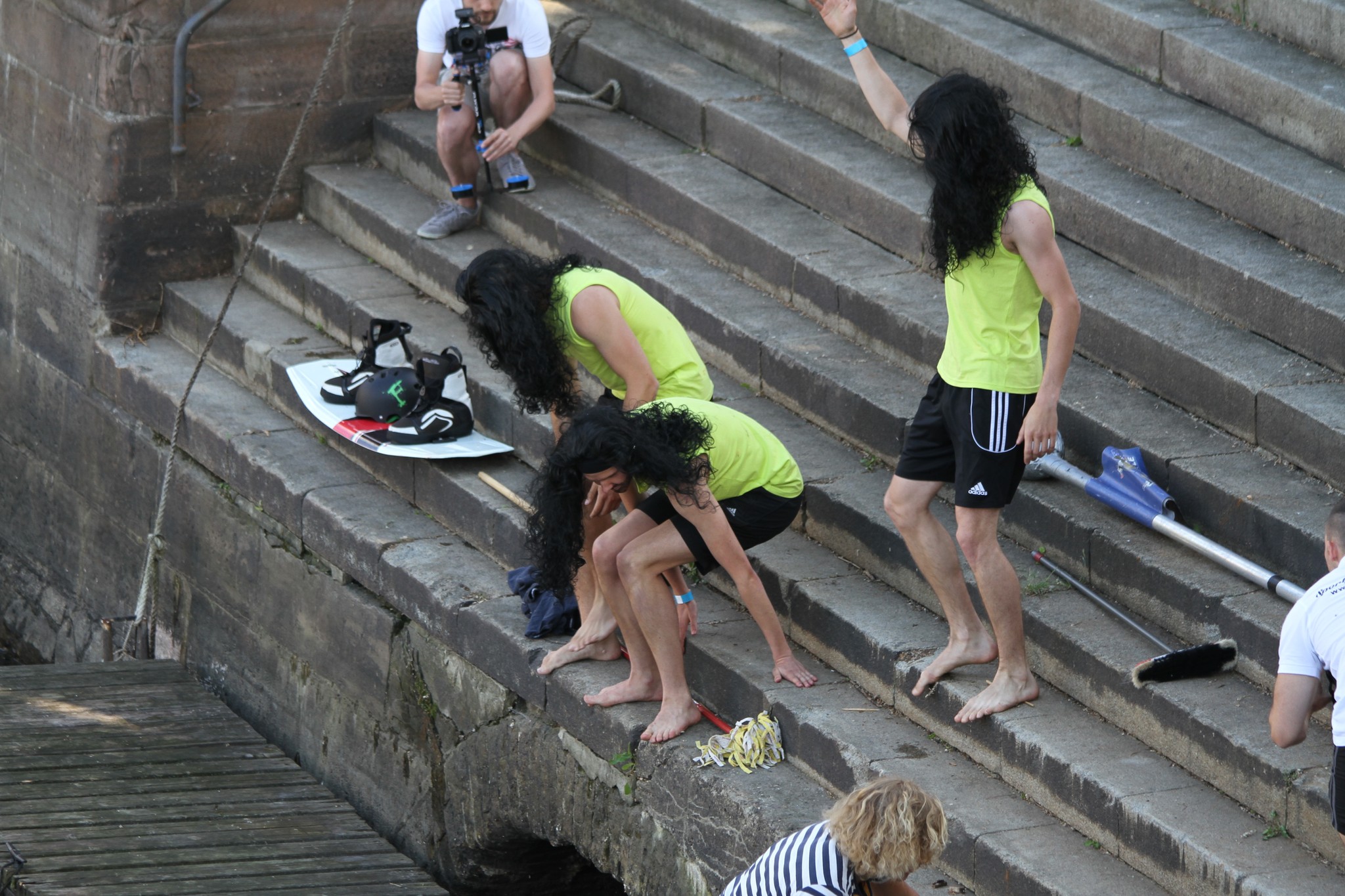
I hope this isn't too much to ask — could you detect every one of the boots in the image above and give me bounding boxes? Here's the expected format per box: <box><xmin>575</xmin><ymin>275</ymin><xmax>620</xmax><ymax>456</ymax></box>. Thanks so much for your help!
<box><xmin>386</xmin><ymin>346</ymin><xmax>474</xmax><ymax>444</ymax></box>
<box><xmin>321</xmin><ymin>319</ymin><xmax>416</xmax><ymax>405</ymax></box>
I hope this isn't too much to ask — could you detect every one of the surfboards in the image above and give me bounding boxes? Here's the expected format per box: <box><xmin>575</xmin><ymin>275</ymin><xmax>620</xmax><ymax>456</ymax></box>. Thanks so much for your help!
<box><xmin>286</xmin><ymin>358</ymin><xmax>515</xmax><ymax>459</ymax></box>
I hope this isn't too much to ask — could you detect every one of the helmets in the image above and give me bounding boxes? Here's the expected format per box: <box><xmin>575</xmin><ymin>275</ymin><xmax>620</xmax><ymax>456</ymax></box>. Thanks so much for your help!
<box><xmin>356</xmin><ymin>367</ymin><xmax>426</xmax><ymax>423</ymax></box>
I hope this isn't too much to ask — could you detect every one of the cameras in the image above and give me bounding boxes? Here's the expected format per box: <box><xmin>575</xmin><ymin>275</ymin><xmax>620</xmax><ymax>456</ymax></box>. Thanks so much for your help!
<box><xmin>445</xmin><ymin>7</ymin><xmax>509</xmax><ymax>55</ymax></box>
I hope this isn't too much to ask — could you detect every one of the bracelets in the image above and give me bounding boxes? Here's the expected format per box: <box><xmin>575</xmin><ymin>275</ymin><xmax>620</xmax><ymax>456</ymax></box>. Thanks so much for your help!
<box><xmin>839</xmin><ymin>27</ymin><xmax>858</xmax><ymax>39</ymax></box>
<box><xmin>843</xmin><ymin>38</ymin><xmax>868</xmax><ymax>57</ymax></box>
<box><xmin>674</xmin><ymin>588</ymin><xmax>693</xmax><ymax>604</ymax></box>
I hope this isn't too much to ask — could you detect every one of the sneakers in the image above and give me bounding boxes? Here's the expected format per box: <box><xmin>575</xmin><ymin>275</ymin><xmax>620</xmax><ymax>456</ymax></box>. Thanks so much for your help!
<box><xmin>416</xmin><ymin>199</ymin><xmax>482</xmax><ymax>239</ymax></box>
<box><xmin>494</xmin><ymin>149</ymin><xmax>535</xmax><ymax>193</ymax></box>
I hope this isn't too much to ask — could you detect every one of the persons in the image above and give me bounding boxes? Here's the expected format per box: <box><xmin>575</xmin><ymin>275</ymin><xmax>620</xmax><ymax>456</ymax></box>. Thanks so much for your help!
<box><xmin>810</xmin><ymin>0</ymin><xmax>1082</xmax><ymax>724</ymax></box>
<box><xmin>414</xmin><ymin>0</ymin><xmax>556</xmax><ymax>239</ymax></box>
<box><xmin>1268</xmin><ymin>495</ymin><xmax>1345</xmax><ymax>846</ymax></box>
<box><xmin>718</xmin><ymin>777</ymin><xmax>948</xmax><ymax>896</ymax></box>
<box><xmin>455</xmin><ymin>250</ymin><xmax>818</xmax><ymax>743</ymax></box>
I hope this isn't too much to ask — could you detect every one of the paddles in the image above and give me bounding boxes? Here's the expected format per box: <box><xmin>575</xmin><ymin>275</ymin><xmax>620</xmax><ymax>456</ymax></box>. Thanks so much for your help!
<box><xmin>478</xmin><ymin>470</ymin><xmax>537</xmax><ymax>515</ymax></box>
